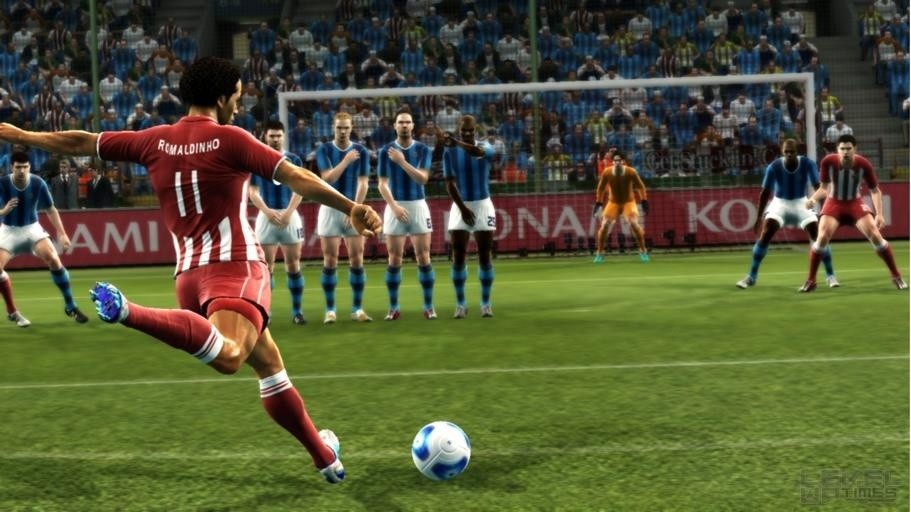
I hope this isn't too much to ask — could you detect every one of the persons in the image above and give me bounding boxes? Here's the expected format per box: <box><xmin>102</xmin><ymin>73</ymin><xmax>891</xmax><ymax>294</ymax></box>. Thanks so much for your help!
<box><xmin>1</xmin><ymin>268</ymin><xmax>31</xmax><ymax>327</ymax></box>
<box><xmin>378</xmin><ymin>106</ymin><xmax>436</xmax><ymax>322</ymax></box>
<box><xmin>735</xmin><ymin>137</ymin><xmax>841</xmax><ymax>290</ymax></box>
<box><xmin>1</xmin><ymin>1</ymin><xmax>910</xmax><ymax>209</ymax></box>
<box><xmin>315</xmin><ymin>112</ymin><xmax>372</xmax><ymax>323</ymax></box>
<box><xmin>435</xmin><ymin>115</ymin><xmax>498</xmax><ymax>320</ymax></box>
<box><xmin>592</xmin><ymin>148</ymin><xmax>649</xmax><ymax>266</ymax></box>
<box><xmin>1</xmin><ymin>152</ymin><xmax>88</xmax><ymax>323</ymax></box>
<box><xmin>248</xmin><ymin>121</ymin><xmax>306</xmax><ymax>326</ymax></box>
<box><xmin>802</xmin><ymin>134</ymin><xmax>909</xmax><ymax>293</ymax></box>
<box><xmin>1</xmin><ymin>58</ymin><xmax>382</xmax><ymax>483</ymax></box>
